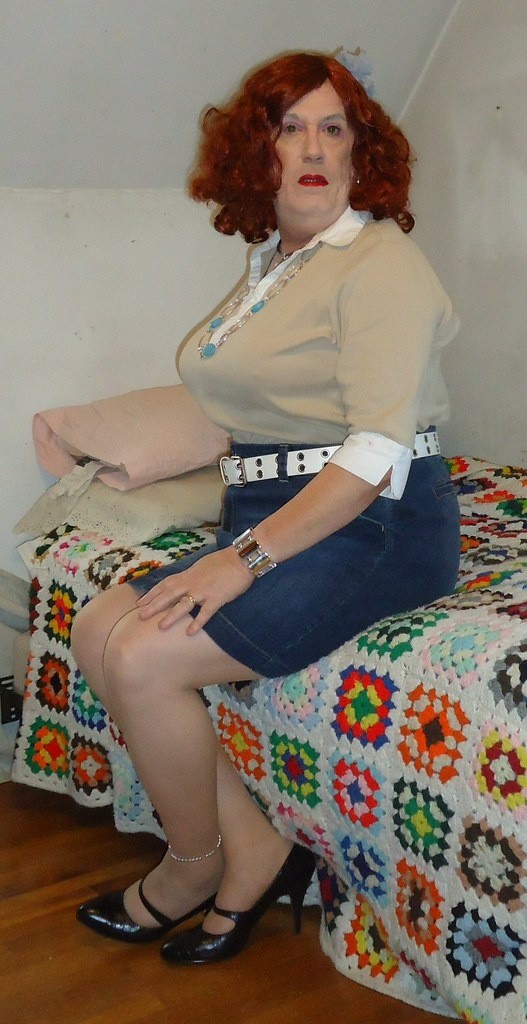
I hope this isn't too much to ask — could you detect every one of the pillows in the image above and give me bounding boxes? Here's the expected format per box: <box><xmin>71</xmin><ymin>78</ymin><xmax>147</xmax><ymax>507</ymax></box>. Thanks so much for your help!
<box><xmin>32</xmin><ymin>383</ymin><xmax>233</xmax><ymax>493</ymax></box>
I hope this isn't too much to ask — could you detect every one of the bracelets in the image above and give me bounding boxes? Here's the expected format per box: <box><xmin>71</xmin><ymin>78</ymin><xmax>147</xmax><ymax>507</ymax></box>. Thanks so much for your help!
<box><xmin>233</xmin><ymin>528</ymin><xmax>277</xmax><ymax>579</ymax></box>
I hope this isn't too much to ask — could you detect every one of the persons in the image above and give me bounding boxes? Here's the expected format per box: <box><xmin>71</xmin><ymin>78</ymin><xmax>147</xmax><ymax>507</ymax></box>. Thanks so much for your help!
<box><xmin>71</xmin><ymin>53</ymin><xmax>461</xmax><ymax>964</ymax></box>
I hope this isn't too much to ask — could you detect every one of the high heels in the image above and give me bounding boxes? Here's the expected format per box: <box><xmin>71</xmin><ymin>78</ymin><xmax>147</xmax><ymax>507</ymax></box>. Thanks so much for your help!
<box><xmin>77</xmin><ymin>873</ymin><xmax>217</xmax><ymax>944</ymax></box>
<box><xmin>161</xmin><ymin>840</ymin><xmax>317</xmax><ymax>965</ymax></box>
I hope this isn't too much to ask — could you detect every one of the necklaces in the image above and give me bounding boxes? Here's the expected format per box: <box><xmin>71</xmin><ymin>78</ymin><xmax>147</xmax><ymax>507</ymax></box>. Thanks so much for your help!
<box><xmin>196</xmin><ymin>241</ymin><xmax>322</xmax><ymax>357</ymax></box>
<box><xmin>276</xmin><ymin>240</ymin><xmax>303</xmax><ymax>266</ymax></box>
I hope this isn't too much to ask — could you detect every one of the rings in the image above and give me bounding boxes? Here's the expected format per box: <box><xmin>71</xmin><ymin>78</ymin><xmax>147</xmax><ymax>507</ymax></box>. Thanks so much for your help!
<box><xmin>187</xmin><ymin>594</ymin><xmax>196</xmax><ymax>604</ymax></box>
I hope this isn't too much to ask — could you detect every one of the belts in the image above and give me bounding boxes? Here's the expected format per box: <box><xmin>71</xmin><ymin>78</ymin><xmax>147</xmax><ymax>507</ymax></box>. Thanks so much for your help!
<box><xmin>218</xmin><ymin>432</ymin><xmax>441</xmax><ymax>487</ymax></box>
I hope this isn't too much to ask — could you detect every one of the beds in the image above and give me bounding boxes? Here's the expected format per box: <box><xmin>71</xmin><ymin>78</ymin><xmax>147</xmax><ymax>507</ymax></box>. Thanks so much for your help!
<box><xmin>0</xmin><ymin>384</ymin><xmax>527</xmax><ymax>1024</ymax></box>
